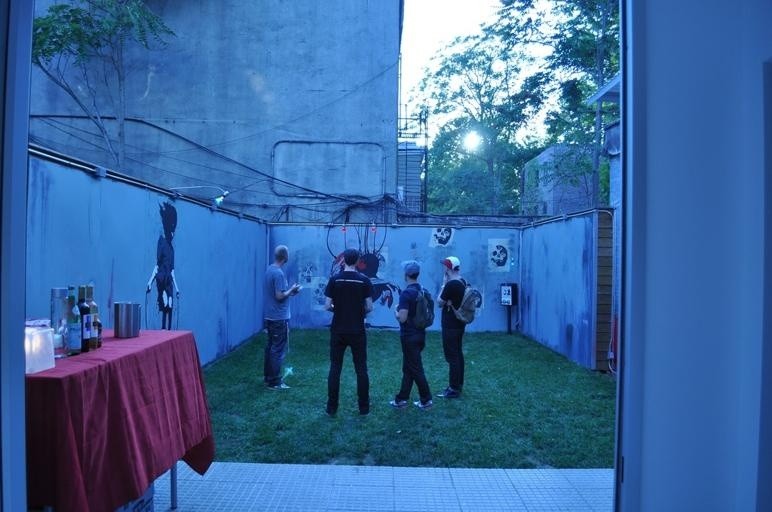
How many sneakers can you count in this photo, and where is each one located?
[435,388,460,400]
[389,399,408,410]
[413,400,434,410]
[358,408,372,418]
[266,380,292,393]
[323,408,339,419]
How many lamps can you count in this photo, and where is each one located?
[170,186,229,206]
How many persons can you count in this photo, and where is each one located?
[436,257,472,398]
[263,245,301,392]
[388,261,433,409]
[324,249,374,418]
[146,202,180,330]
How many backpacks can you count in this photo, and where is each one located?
[445,278,480,324]
[407,284,434,331]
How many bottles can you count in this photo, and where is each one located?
[51,283,102,357]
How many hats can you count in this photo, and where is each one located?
[441,256,460,271]
[400,260,421,274]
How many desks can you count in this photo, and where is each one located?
[25,329,215,512]
